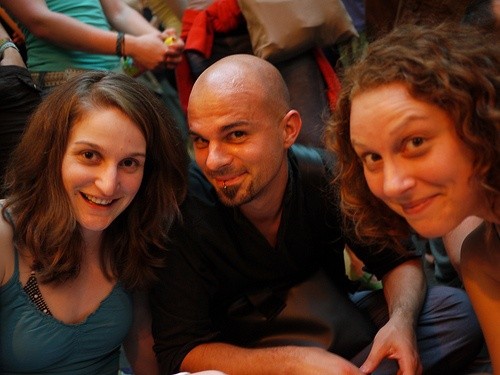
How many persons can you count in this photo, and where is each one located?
[322,21,499,375]
[0,70,187,375]
[0,0,500,375]
[151,53,493,375]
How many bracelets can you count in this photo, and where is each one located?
[116,32,123,58]
[122,33,127,60]
[0,42,19,56]
[0,37,14,46]
